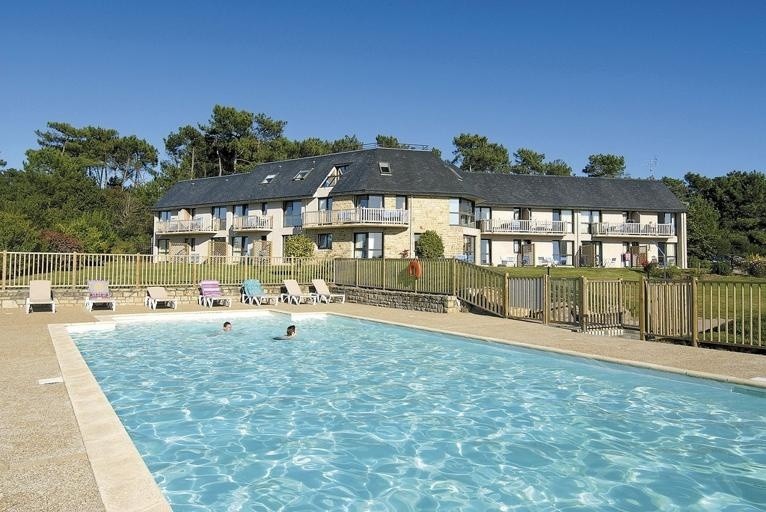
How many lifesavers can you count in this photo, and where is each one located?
[408,262,421,280]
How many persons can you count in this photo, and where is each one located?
[214,322,232,335]
[278,325,296,339]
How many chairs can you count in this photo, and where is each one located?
[24,279,55,314]
[83,278,347,312]
